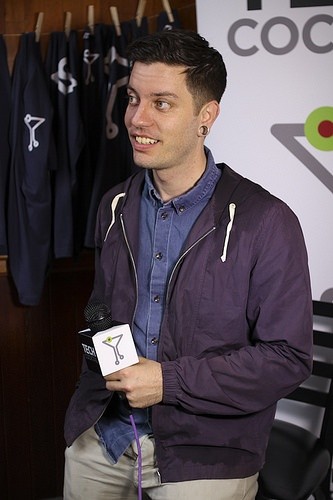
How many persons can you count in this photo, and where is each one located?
[63,29,314,500]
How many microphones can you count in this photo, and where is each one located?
[78,300,140,416]
